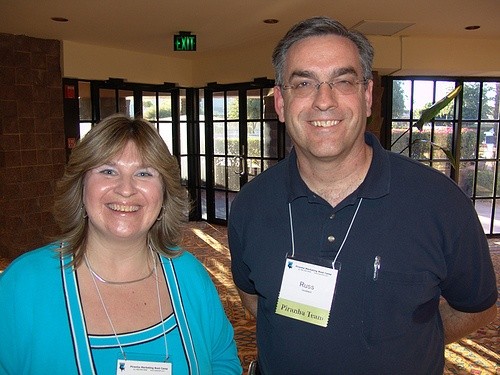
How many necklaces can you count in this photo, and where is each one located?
[81,251,157,285]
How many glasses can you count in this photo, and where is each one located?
[279,77,368,98]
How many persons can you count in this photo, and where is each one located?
[228,15,498,375]
[0,114,247,375]
